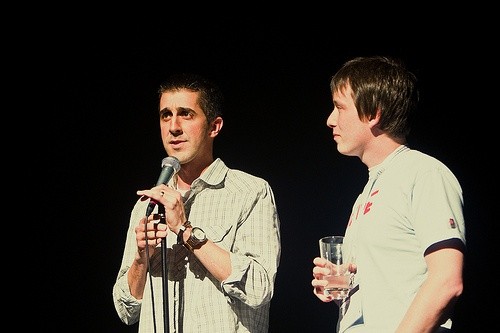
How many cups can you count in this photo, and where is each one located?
[318,236,351,299]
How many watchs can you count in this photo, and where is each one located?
[182,227,207,250]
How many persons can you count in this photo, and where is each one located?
[111,70,282,333]
[310,56,465,333]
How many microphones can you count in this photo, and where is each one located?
[145,156,182,216]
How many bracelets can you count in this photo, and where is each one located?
[177,221,191,245]
